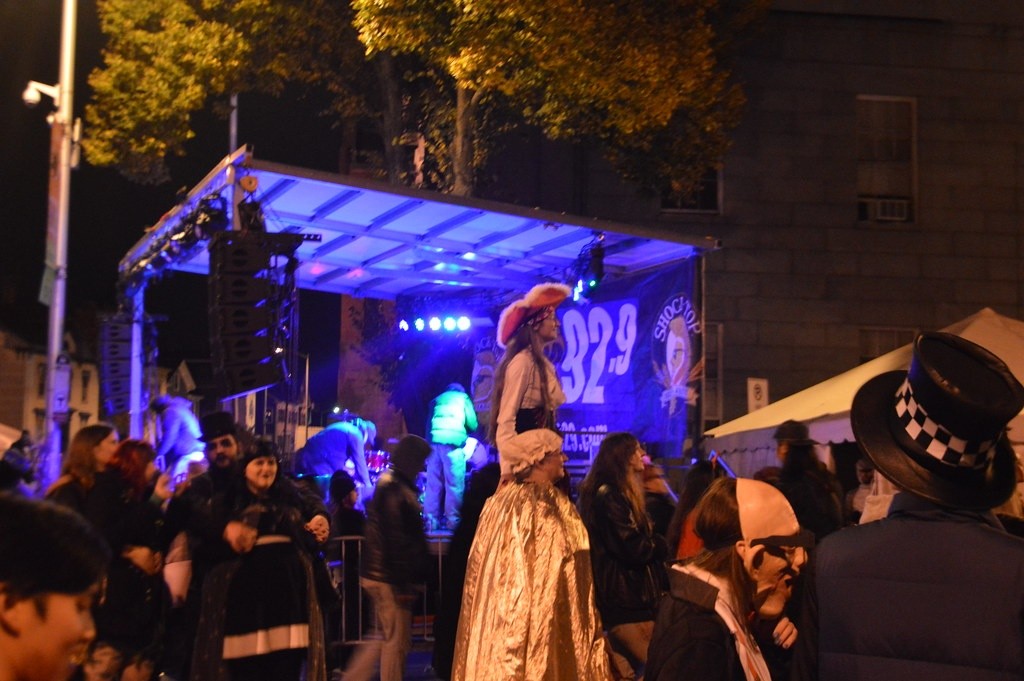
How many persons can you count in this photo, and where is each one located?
[452,428,615,681]
[577,419,842,681]
[794,331,1024,681]
[422,382,488,531]
[0,398,376,681]
[643,477,808,681]
[486,284,574,496]
[341,433,434,681]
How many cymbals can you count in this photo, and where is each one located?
[325,412,358,419]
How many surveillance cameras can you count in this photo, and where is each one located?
[23,88,40,109]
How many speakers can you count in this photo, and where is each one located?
[95,321,133,416]
[207,231,284,402]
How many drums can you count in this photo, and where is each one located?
[364,449,390,474]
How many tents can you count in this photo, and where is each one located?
[705,306,1023,478]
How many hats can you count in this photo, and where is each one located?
[851,331,1024,510]
[773,420,821,447]
[238,441,286,462]
[498,284,574,348]
[195,411,242,442]
[329,477,356,499]
[501,428,562,475]
